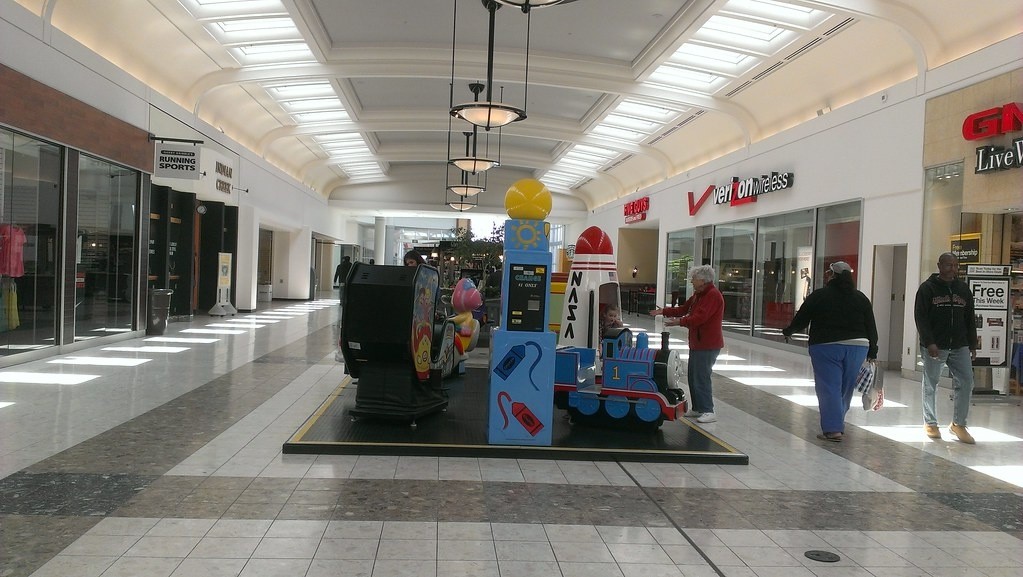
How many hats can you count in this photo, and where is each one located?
[830,261,853,274]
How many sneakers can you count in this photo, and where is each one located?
[685,410,702,417]
[949,422,975,444]
[697,412,716,423]
[923,424,941,438]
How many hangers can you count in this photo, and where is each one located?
[0,278,18,287]
[0,216,18,226]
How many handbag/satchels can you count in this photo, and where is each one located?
[855,359,884,410]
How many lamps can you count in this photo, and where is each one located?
[936,167,944,180]
[445,160,479,212]
[943,165,952,178]
[494,0,577,9]
[952,164,959,177]
[631,265,638,279]
[446,128,487,197]
[446,83,504,175]
[447,0,530,129]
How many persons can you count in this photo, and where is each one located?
[599,305,624,356]
[334,255,352,308]
[914,252,978,445]
[782,261,879,442]
[649,265,725,424]
[670,275,683,308]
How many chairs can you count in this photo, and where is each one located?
[556,346,598,388]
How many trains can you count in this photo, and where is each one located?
[555,327,688,430]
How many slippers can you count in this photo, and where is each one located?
[817,434,840,442]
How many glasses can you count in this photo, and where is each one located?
[942,262,961,269]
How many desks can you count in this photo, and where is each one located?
[639,288,656,312]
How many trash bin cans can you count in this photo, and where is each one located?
[146,289,174,335]
